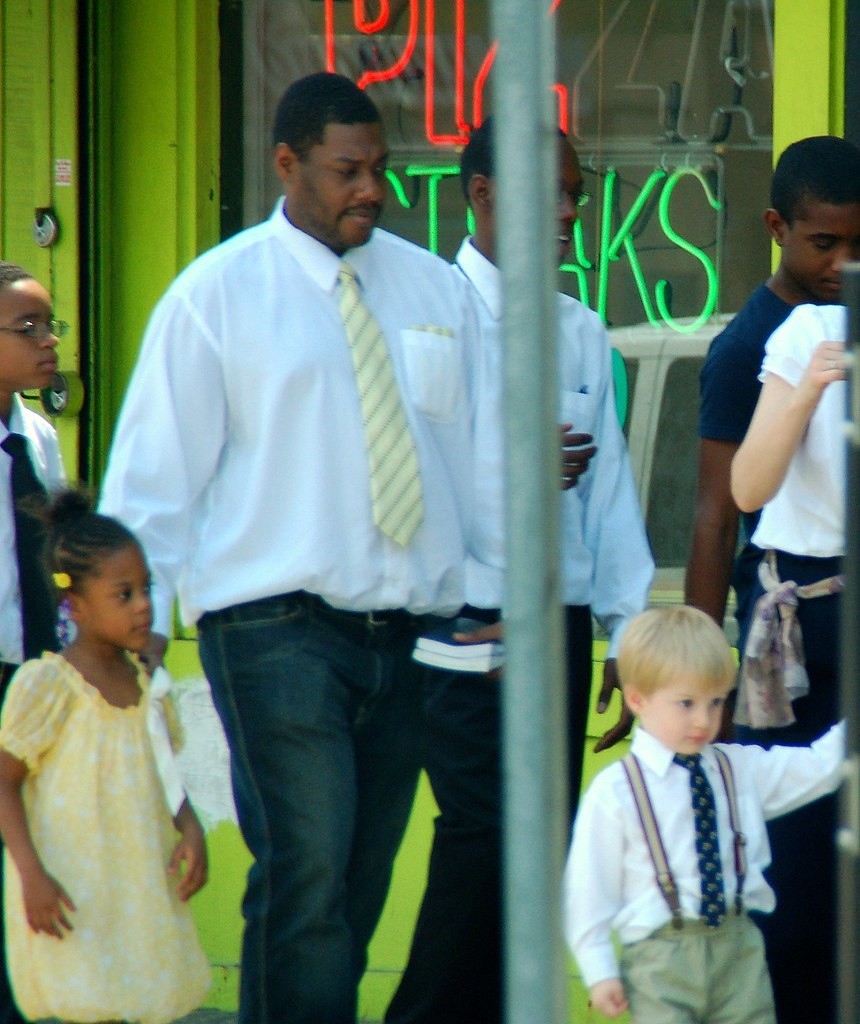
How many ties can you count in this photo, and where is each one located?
[337,261,424,548]
[0,433,64,662]
[672,752,726,931]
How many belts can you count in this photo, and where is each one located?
[268,589,412,626]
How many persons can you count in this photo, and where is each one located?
[0,490,213,1024]
[565,606,845,1024]
[728,303,849,1024]
[675,135,860,654]
[0,258,78,710]
[382,113,657,1024]
[94,73,505,1024]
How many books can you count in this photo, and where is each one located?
[412,617,505,674]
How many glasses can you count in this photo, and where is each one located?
[0,320,70,338]
[557,188,593,207]
